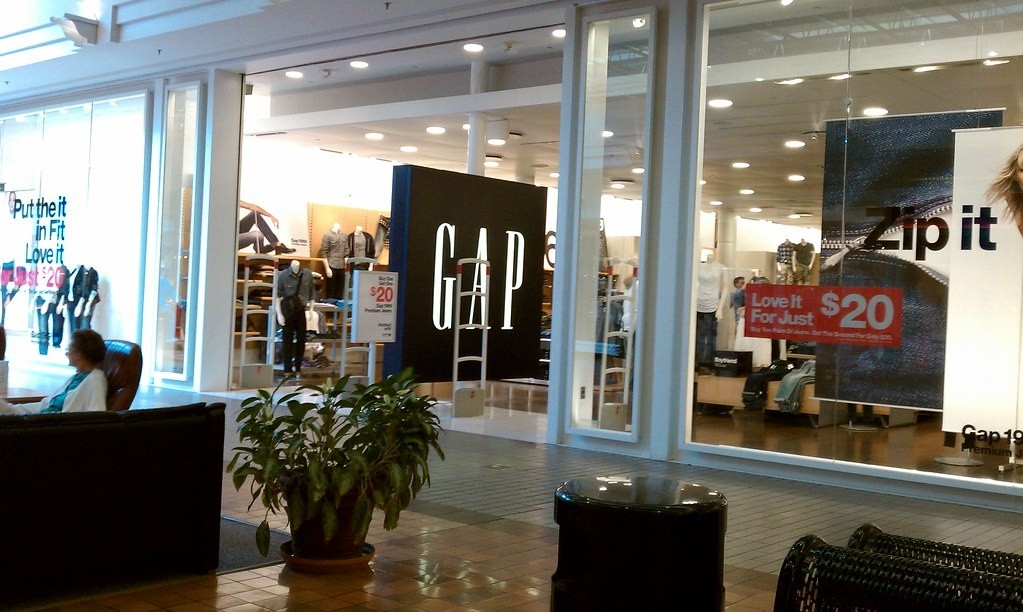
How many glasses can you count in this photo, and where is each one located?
[66,347,70,352]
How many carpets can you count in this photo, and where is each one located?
[22,515,292,612]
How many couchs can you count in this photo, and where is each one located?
[0,401,226,612]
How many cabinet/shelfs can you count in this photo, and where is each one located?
[500,337,551,386]
[543,268,619,317]
[234,252,389,349]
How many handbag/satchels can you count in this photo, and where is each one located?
[276,273,306,326]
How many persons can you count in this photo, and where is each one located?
[347,225,376,287]
[35,262,100,355]
[792,238,816,287]
[239,200,296,254]
[0,329,107,411]
[777,239,796,286]
[275,259,316,375]
[321,224,350,324]
[732,273,755,338]
[696,254,729,373]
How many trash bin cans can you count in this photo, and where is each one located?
[550,474,728,612]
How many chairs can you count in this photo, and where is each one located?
[94,340,143,411]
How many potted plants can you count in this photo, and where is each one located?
[224,365,446,575]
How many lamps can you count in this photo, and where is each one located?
[486,119,510,145]
